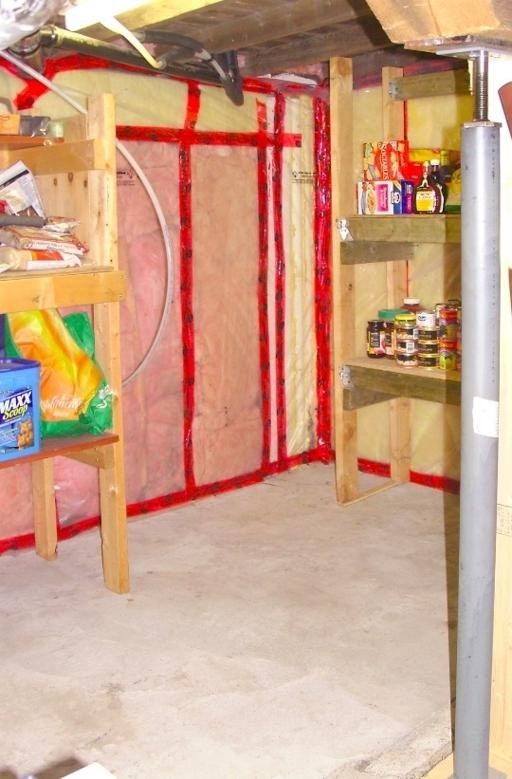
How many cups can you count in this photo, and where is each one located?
[46,121,64,145]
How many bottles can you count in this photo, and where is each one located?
[415,149,454,214]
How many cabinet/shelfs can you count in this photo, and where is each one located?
[0,91,130,594]
[329,56,475,507]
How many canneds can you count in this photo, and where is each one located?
[366,304,462,367]
